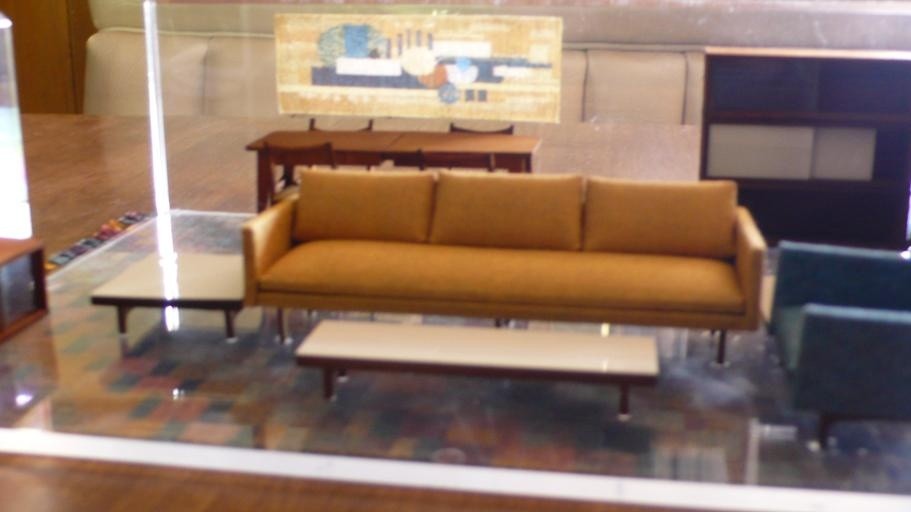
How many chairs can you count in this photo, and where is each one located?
[768,240,911,450]
[244,119,543,214]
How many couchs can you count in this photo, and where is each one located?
[240,170,768,372]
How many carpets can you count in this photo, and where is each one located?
[1,210,911,493]
